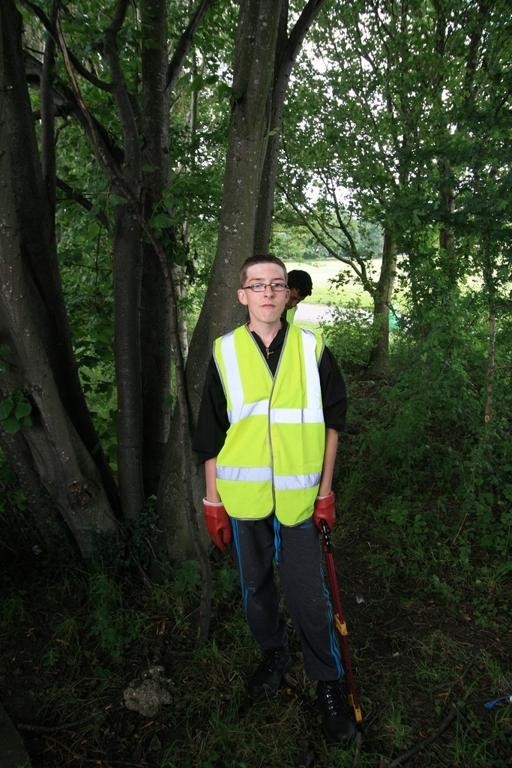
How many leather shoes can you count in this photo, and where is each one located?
[248,645,294,702]
[315,680,355,744]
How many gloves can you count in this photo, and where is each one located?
[203,496,232,551]
[313,491,337,533]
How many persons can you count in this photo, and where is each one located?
[190,252,359,747]
[285,267,314,324]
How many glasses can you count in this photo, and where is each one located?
[242,282,289,292]
